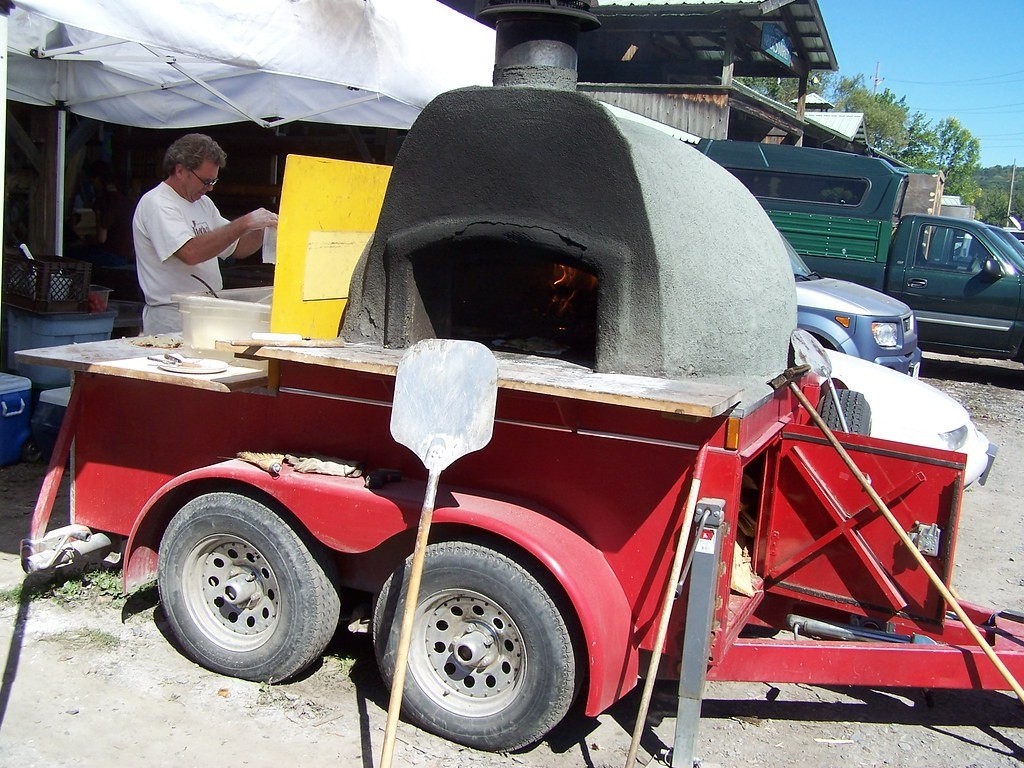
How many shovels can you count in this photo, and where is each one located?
[368,334,500,768]
[789,326,851,433]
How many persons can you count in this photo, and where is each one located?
[131,133,278,335]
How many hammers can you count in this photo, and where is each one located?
[767,362,1024,722]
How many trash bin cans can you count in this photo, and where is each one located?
[5,309,120,404]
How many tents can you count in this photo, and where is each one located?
[7,1,496,129]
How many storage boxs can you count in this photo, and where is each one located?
[6,307,118,384]
[2,254,92,315]
[31,387,70,469]
[0,371,44,466]
[171,286,272,367]
[91,284,114,313]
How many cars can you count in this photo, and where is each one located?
[775,221,922,383]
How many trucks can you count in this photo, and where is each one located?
[695,137,1024,365]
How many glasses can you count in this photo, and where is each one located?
[187,166,219,186]
